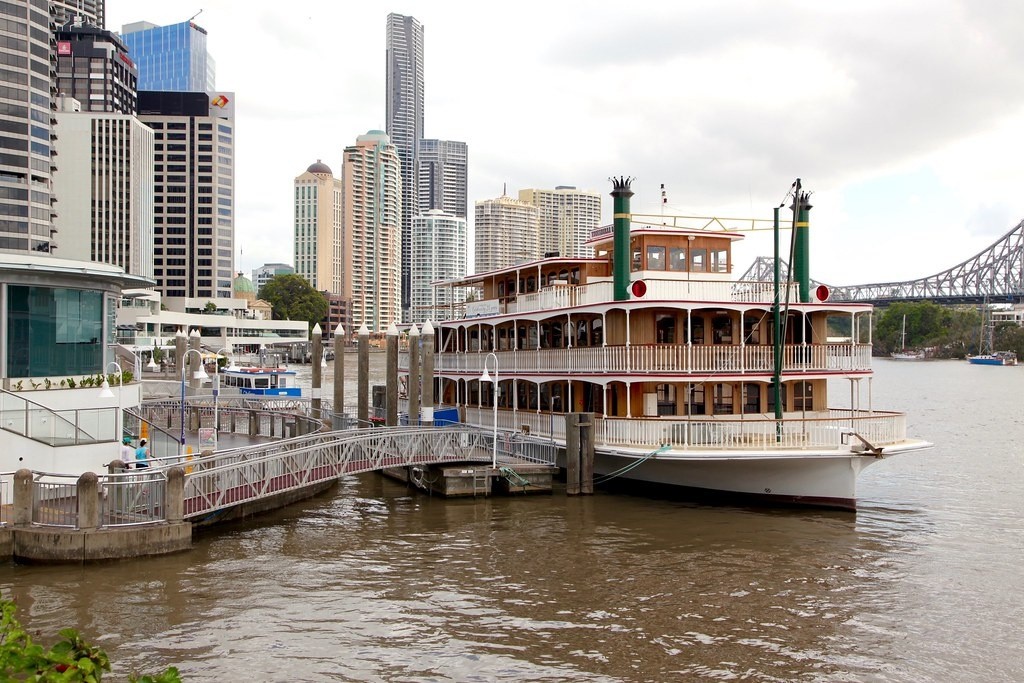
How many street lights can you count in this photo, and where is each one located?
[478,352,499,470]
[212,347,241,452]
[137,345,158,441]
[97,361,123,462]
[179,348,210,461]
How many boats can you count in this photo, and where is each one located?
[270,340,334,363]
[362,173,938,517]
[219,347,303,411]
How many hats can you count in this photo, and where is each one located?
[141,438,146,443]
[123,438,131,443]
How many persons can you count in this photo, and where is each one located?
[122,438,133,469]
[134,438,152,494]
[499,390,559,410]
